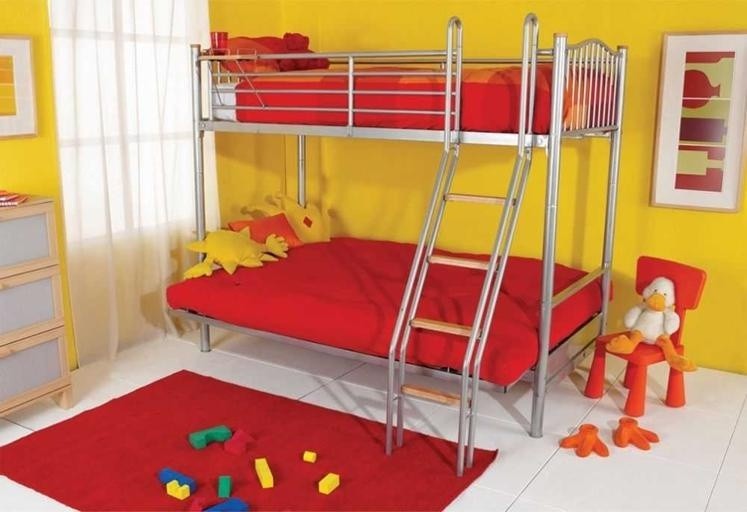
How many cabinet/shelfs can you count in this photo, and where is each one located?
[0,192,79,426]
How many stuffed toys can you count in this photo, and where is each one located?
[603,273,695,374]
[557,423,609,457]
[183,225,288,281]
[241,190,333,244]
[611,417,659,450]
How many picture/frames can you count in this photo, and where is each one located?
[1,32,38,141]
[649,32,747,215]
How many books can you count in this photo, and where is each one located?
[0,189,31,207]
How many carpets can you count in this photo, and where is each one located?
[0,370,496,512]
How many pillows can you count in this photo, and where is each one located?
[183,197,334,278]
[218,31,329,71]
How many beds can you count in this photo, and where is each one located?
[164,13,628,477]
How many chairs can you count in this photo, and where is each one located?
[583,255,707,416]
